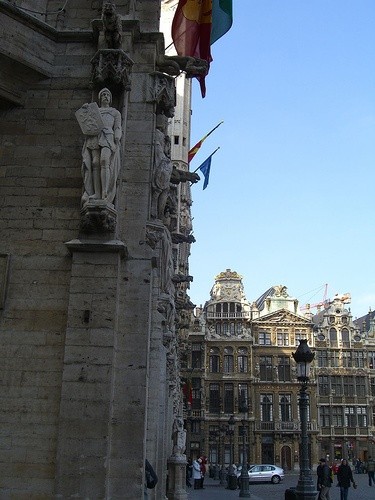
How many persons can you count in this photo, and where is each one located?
[317,458,332,500]
[352,457,367,474]
[366,456,375,487]
[336,459,355,500]
[75,87,122,201]
[186,456,208,489]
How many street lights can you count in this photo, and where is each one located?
[292,338,320,500]
[237,388,251,498]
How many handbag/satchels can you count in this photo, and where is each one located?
[353,483,357,489]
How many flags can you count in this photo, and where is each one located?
[171,0,233,97]
[188,120,224,164]
[183,373,193,408]
[193,147,220,190]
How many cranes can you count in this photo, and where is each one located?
[299,282,352,311]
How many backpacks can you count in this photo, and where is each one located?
[328,467,334,483]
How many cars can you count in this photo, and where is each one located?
[237,463,285,484]
